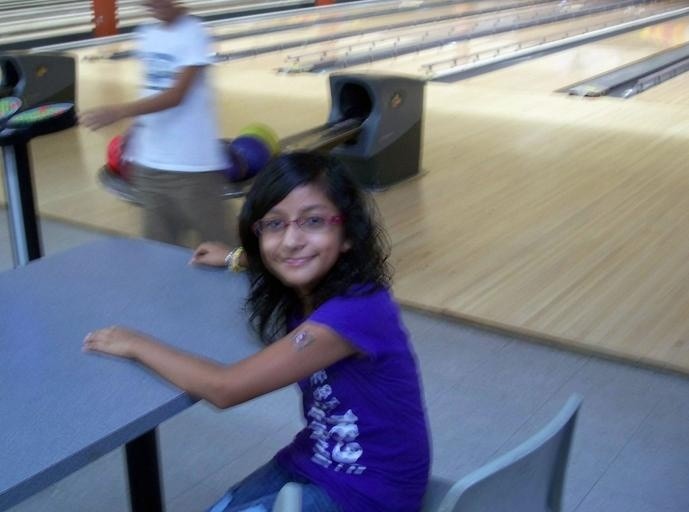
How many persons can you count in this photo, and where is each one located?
[73,0,230,256]
[81,150,430,512]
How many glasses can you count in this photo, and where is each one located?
[253,214,341,238]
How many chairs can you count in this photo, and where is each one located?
[382,390,581,511]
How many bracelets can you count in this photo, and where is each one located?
[224,246,249,273]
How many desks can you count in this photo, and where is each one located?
[0,229,309,512]
